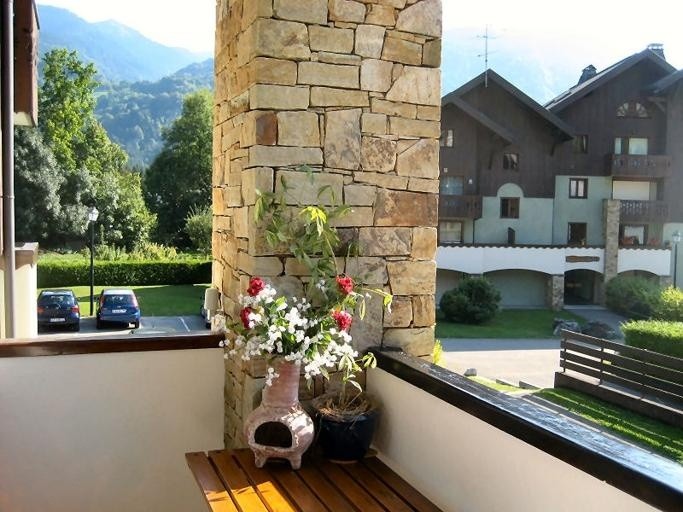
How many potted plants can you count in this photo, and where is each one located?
[254,165,393,462]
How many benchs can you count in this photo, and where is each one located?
[185,449,444,512]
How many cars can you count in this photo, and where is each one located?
[37,288,80,333]
[92,288,141,329]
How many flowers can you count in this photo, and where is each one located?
[209,275,359,387]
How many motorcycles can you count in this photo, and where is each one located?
[199,296,204,318]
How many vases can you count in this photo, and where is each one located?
[243,356,315,471]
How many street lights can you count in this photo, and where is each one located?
[88,207,100,319]
[672,229,681,290]
